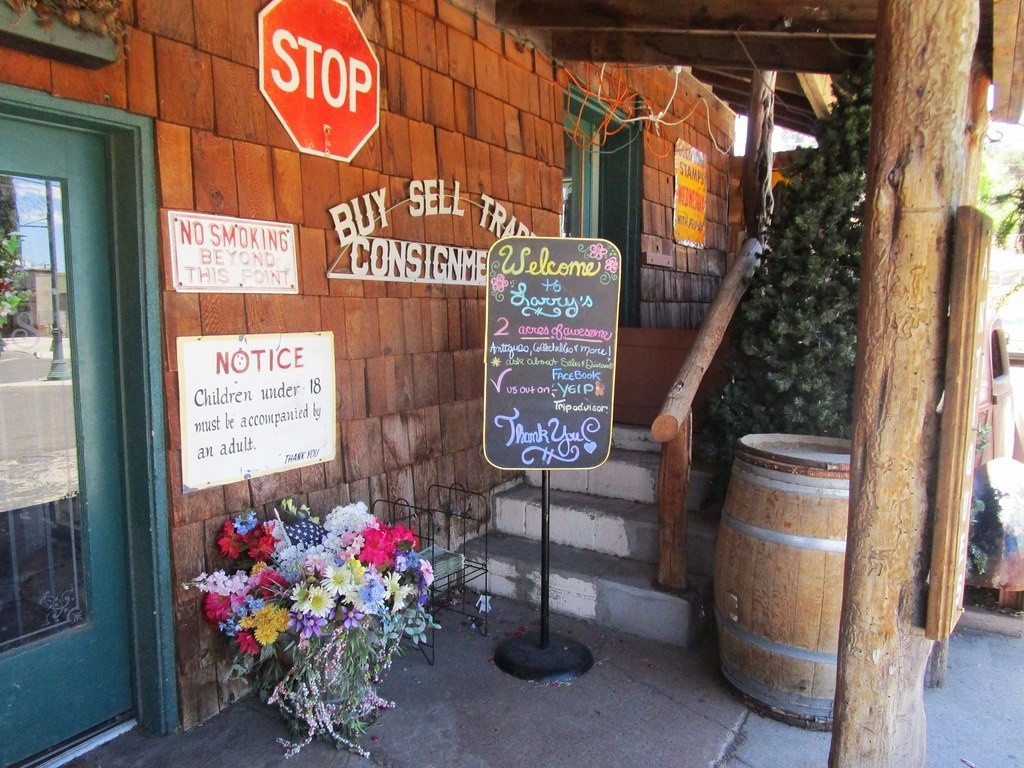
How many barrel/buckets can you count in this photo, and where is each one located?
[715,431,851,729]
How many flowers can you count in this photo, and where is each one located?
[180,498,442,760]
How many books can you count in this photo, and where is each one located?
[415,543,465,581]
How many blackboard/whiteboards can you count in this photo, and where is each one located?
[482,236,622,471]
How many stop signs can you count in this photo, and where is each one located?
[256,0,380,164]
